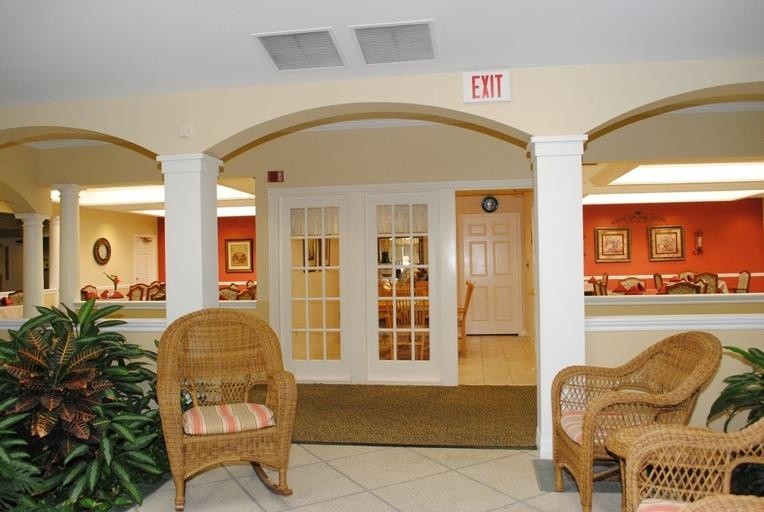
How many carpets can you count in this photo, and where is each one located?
[291,383,537,450]
[533,459,622,493]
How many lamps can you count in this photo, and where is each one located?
[693,232,703,256]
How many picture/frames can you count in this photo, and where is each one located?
[224,238,254,274]
[303,239,330,273]
[378,236,423,265]
[595,226,632,263]
[648,224,686,261]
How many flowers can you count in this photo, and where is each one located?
[103,272,120,290]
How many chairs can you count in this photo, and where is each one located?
[157,307,298,512]
[551,331,722,512]
[583,270,751,296]
[376,280,474,361]
[625,417,763,511]
[0,280,258,304]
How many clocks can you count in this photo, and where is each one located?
[481,196,498,213]
[94,238,111,265]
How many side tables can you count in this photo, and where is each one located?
[605,423,726,512]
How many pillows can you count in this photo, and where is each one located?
[182,403,275,435]
